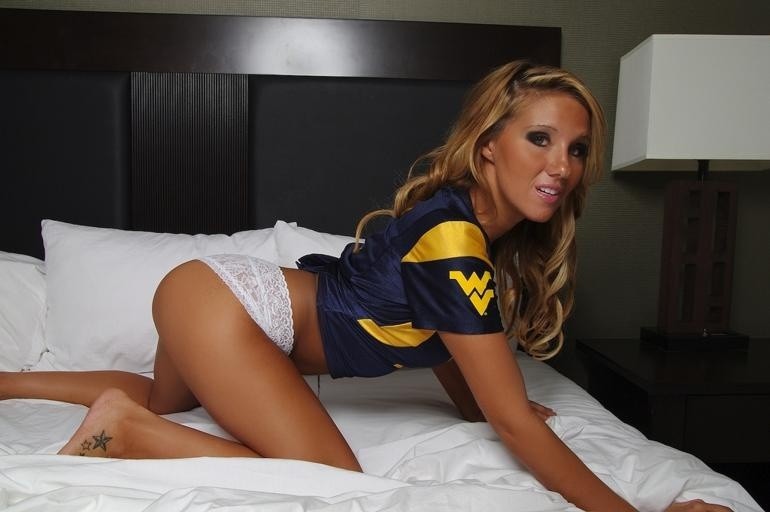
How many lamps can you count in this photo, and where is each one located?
[611,33,770,352]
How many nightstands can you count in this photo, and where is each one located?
[575,334,770,512]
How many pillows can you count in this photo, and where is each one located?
[37,217,297,374]
[1,250,46,371]
[270,220,370,272]
